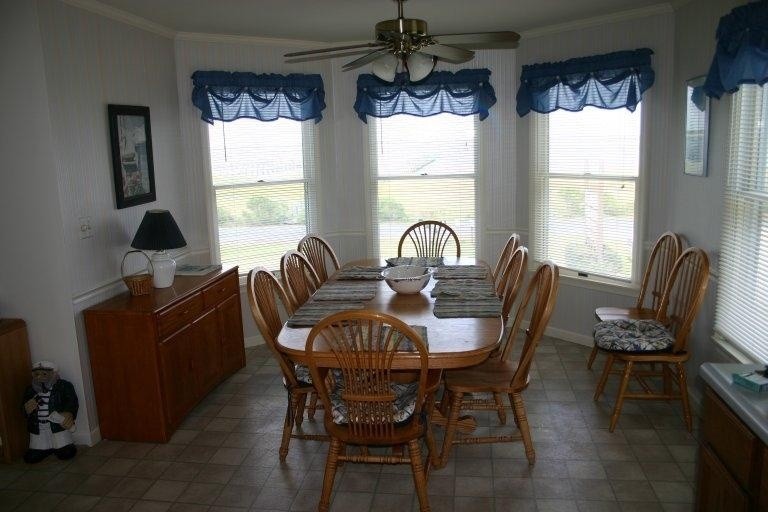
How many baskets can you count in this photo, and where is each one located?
[120,250,156,298]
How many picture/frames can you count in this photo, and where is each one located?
[108,104,156,210]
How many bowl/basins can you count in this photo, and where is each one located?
[380,264,434,294]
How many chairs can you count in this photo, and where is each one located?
[492,234,521,286]
[396,220,462,261]
[298,234,342,291]
[496,246,528,319]
[595,246,711,434]
[587,230,684,402]
[279,248,332,421]
[305,309,440,511]
[247,264,420,465]
[441,261,560,467]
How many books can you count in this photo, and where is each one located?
[175,265,221,276]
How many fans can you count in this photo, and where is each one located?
[284,0,520,74]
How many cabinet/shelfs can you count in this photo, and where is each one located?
[698,361,768,512]
[83,265,247,444]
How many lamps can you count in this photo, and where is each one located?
[372,0,437,85]
[131,208,188,289]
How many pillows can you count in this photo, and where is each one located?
[592,318,675,353]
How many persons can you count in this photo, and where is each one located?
[18,362,79,463]
